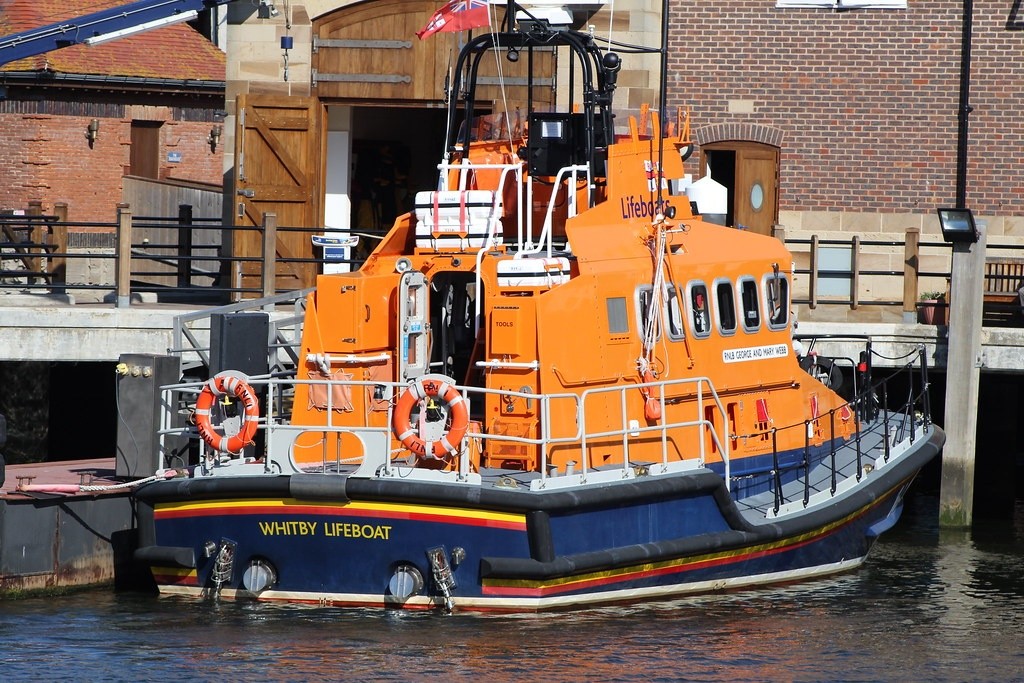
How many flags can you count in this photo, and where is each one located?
[415,0,492,41]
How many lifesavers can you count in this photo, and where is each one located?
[194,376,260,454]
[392,379,470,459]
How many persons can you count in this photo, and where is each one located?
[1018,276,1024,314]
[465,287,484,353]
[351,144,435,245]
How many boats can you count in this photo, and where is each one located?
[121,0,949,618]
